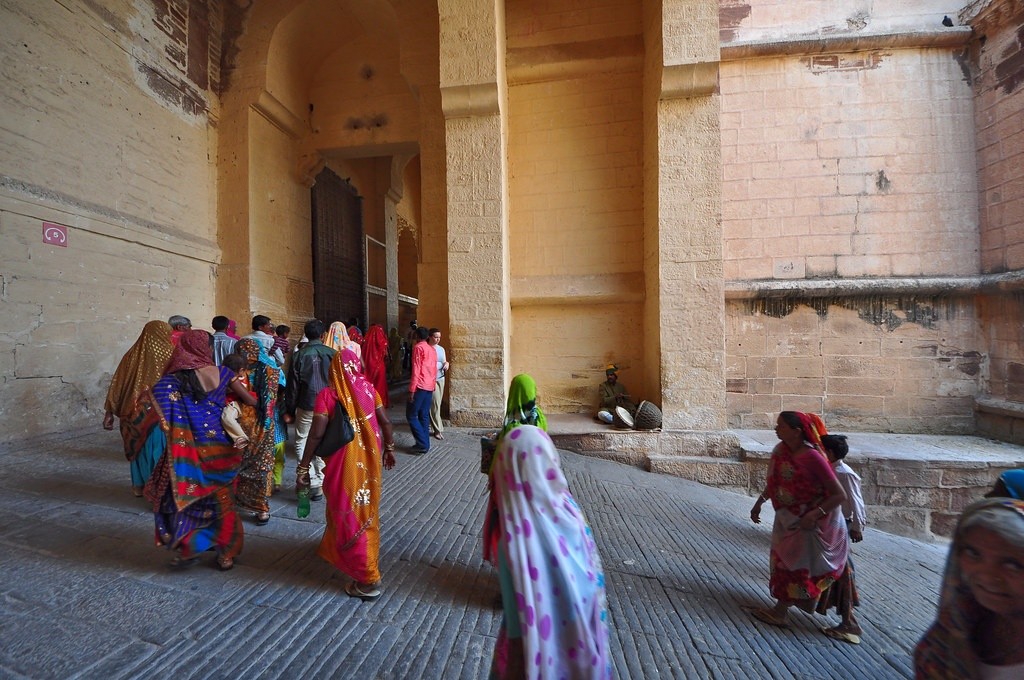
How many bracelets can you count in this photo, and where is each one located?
[761,495,766,502]
[385,443,394,451]
[818,507,826,514]
[296,464,311,474]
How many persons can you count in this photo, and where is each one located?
[487,425,614,680]
[482,375,547,566]
[296,349,396,573]
[233,337,281,526]
[406,326,438,453]
[103,320,174,496]
[325,321,417,408]
[212,315,309,492]
[221,354,251,449]
[750,411,866,644]
[984,469,1024,500]
[912,496,1024,680]
[168,316,192,345]
[597,365,636,423]
[287,319,339,501]
[427,328,450,439]
[143,328,256,570]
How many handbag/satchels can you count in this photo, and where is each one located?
[313,392,354,458]
[479,434,499,473]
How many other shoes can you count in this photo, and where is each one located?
[216,554,233,570]
[308,486,324,502]
[274,483,281,493]
[169,553,202,569]
[131,486,144,496]
[407,447,428,455]
[433,431,445,440]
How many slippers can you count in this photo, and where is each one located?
[821,626,861,644]
[753,608,793,628]
[254,510,270,525]
[344,580,380,599]
